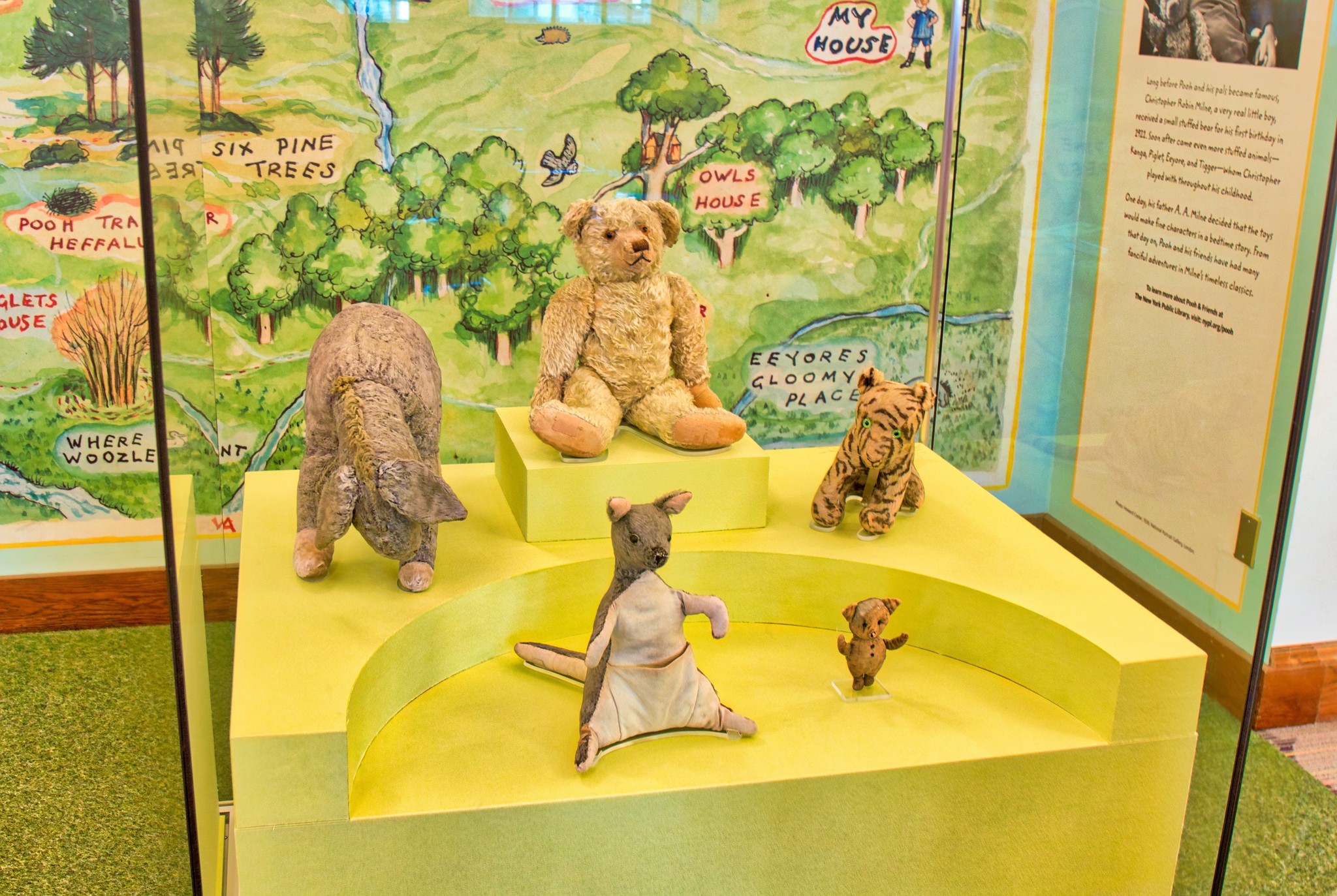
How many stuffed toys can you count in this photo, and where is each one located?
[810,366,934,534]
[527,195,744,456]
[838,597,908,690]
[294,303,468,593]
[512,490,759,771]
